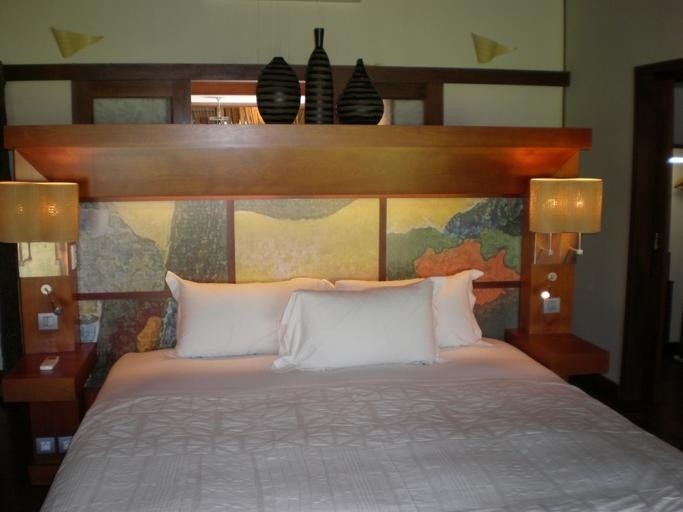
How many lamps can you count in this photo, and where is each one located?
[666,147,682,164]
[1,183,81,267]
[40,284,63,315]
[528,177,606,265]
[539,272,558,301]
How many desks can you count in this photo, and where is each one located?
[502,331,611,382]
[0,341,99,486]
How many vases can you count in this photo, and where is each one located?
[303,27,334,124]
[336,58,385,125]
[255,57,301,124]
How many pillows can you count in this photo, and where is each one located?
[272,276,442,371]
[334,267,485,351]
[163,269,336,361]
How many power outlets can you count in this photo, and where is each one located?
[58,434,73,456]
[33,437,54,456]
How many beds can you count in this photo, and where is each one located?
[39,338,683,512]
